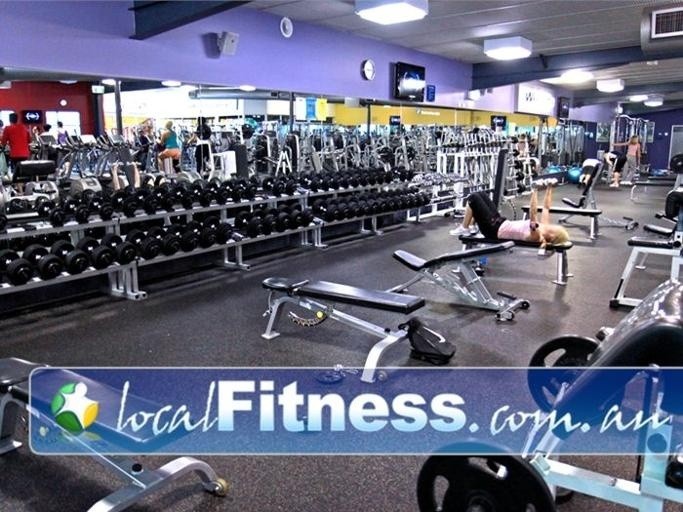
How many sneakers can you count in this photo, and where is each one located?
[449,224,472,237]
[609,178,633,188]
[468,224,478,234]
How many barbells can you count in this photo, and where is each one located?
[442,132,538,196]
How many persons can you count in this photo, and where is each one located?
[1,113,32,198]
[38,124,56,145]
[56,121,66,145]
[135,117,153,171]
[612,134,640,182]
[154,120,181,172]
[601,151,627,188]
[578,158,601,189]
[449,178,570,243]
[190,117,211,181]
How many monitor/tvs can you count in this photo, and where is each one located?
[0,110,16,129]
[558,97,570,118]
[45,111,80,139]
[394,61,426,102]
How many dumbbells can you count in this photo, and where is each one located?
[414,171,467,188]
[0,166,431,287]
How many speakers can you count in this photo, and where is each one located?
[215,30,240,56]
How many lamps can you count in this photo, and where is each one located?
[354,0,429,26]
[482,36,533,63]
[643,96,663,107]
[595,79,625,93]
